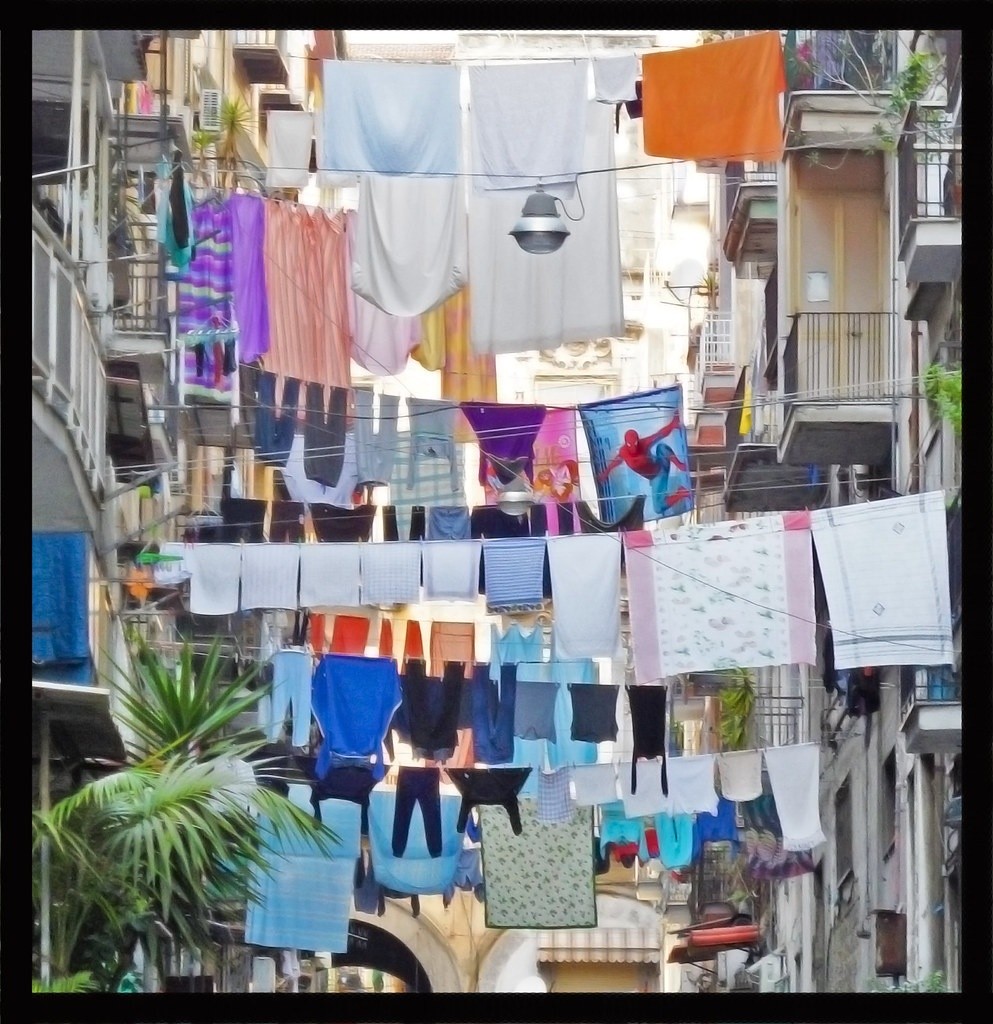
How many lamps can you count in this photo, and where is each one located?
[507,177,572,256]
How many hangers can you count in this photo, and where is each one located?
[186,302,239,346]
[136,524,185,572]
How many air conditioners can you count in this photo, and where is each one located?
[200,88,228,132]
